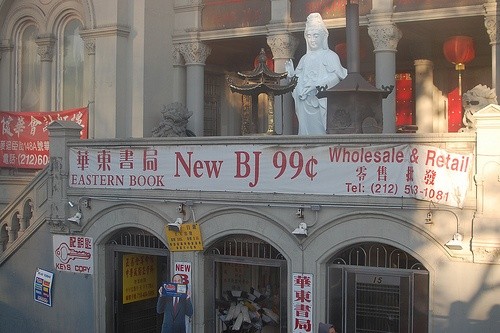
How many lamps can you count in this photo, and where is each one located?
[291,223,307,237]
[168,217,183,232]
[67,212,81,224]
[445,233,463,250]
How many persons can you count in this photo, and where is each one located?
[284,12,349,137]
[156,274,194,333]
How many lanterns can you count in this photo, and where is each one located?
[252,51,275,72]
[331,34,367,69]
[440,33,476,98]
